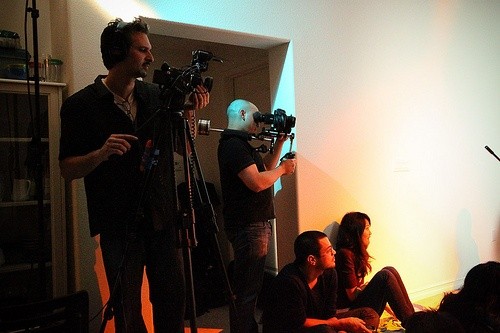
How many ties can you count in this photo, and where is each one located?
[122,100,135,122]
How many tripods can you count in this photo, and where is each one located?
[99,97,242,333]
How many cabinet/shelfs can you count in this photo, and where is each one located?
[0,78,68,324]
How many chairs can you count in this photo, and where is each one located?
[0,289,90,333]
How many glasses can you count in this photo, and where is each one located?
[319,246,333,258]
[115,20,130,32]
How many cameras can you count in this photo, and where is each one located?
[153,50,224,97]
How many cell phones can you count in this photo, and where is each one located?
[366,325,376,333]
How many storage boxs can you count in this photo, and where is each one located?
[0,58,29,79]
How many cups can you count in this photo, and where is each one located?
[12,178,31,201]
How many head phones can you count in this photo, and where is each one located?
[110,22,128,64]
